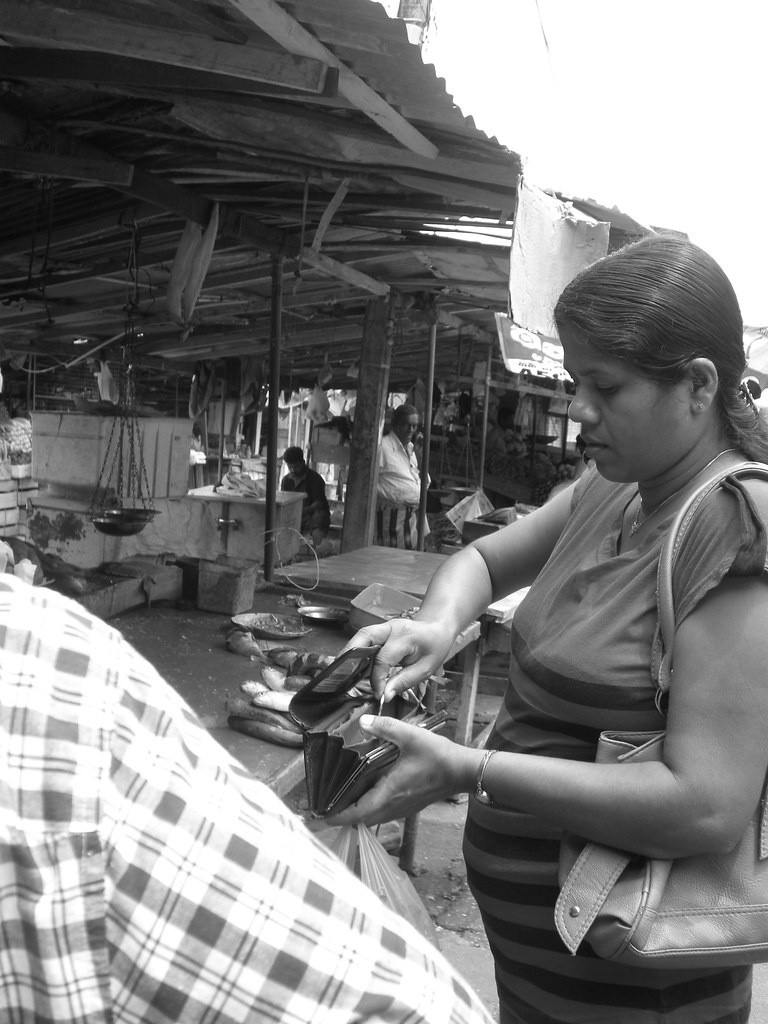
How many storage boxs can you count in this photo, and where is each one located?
[196,553,259,615]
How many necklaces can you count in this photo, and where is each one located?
[629,449,737,535]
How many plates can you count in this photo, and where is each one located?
[297,605,350,625]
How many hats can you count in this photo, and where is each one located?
[284,447,303,464]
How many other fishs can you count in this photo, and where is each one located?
[221,628,335,746]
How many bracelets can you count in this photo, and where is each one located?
[475,750,497,808]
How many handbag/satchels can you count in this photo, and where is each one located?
[554,450,768,966]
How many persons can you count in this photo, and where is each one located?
[325,233,767,1024]
[0,571,500,1023]
[280,445,331,554]
[374,403,433,552]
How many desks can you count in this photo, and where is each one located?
[188,485,308,570]
[105,586,482,877]
[274,544,532,804]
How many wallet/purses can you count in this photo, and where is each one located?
[289,644,447,819]
